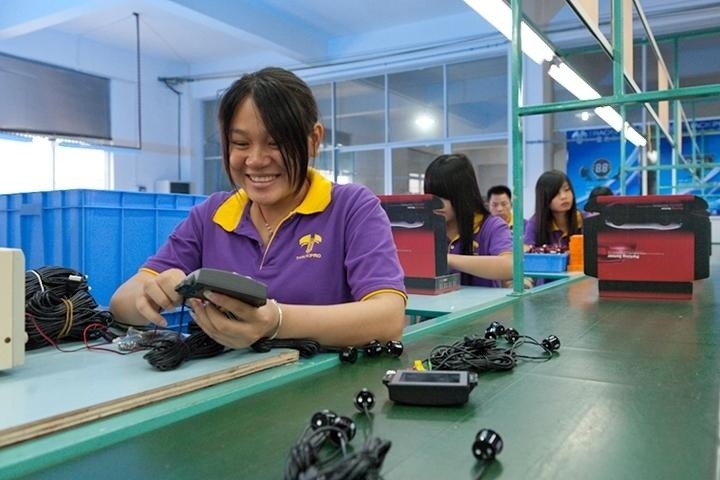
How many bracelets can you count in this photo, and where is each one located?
[266,298,283,341]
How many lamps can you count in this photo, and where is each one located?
[593,106,623,132]
[624,125,648,147]
[547,63,601,100]
[463,0,557,66]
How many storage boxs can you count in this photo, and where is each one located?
[1,191,207,335]
[523,251,568,273]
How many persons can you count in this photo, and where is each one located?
[108,67,408,348]
[424,153,613,289]
